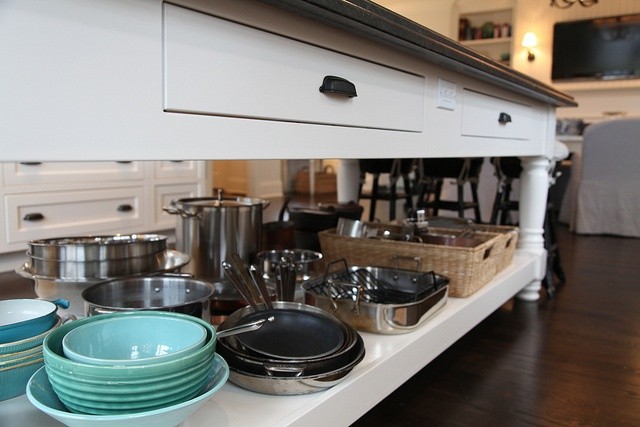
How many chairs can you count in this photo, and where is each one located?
[490,152,575,300]
[357,159,411,218]
[416,157,484,223]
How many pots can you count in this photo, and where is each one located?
[162,186,261,291]
[255,249,323,292]
[81,273,215,311]
[217,261,366,396]
[257,195,295,244]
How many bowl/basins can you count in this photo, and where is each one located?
[15,233,195,319]
[0,298,62,402]
[26,311,227,427]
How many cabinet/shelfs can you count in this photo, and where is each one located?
[0,0,578,427]
[456,7,513,45]
[3,160,205,258]
